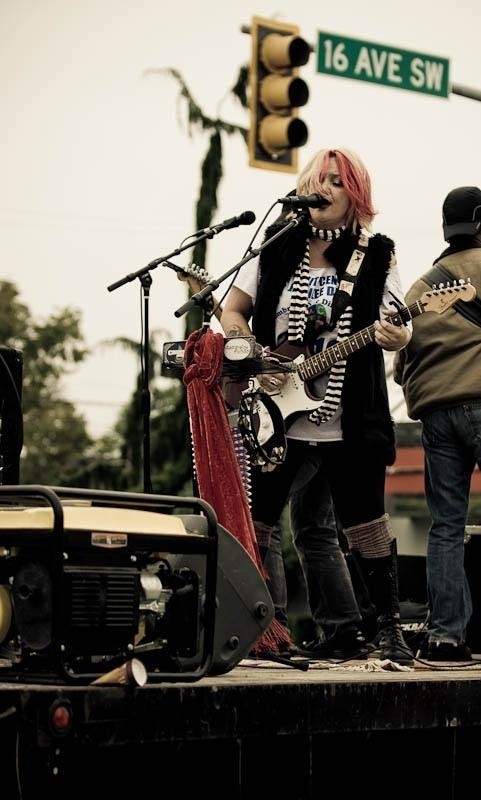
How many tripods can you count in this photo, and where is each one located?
[175,213,309,670]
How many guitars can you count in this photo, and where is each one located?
[217,278,476,459]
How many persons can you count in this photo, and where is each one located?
[392,184,481,661]
[262,186,371,664]
[219,148,417,667]
[221,374,371,661]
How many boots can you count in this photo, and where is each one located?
[348,538,415,666]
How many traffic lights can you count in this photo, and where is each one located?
[246,14,314,176]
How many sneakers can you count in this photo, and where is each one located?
[427,634,458,649]
[295,624,367,660]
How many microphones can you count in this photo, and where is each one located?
[278,193,324,208]
[195,211,255,238]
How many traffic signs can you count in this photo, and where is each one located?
[316,27,453,100]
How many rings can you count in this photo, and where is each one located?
[270,377,279,385]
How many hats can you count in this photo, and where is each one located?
[442,187,480,241]
